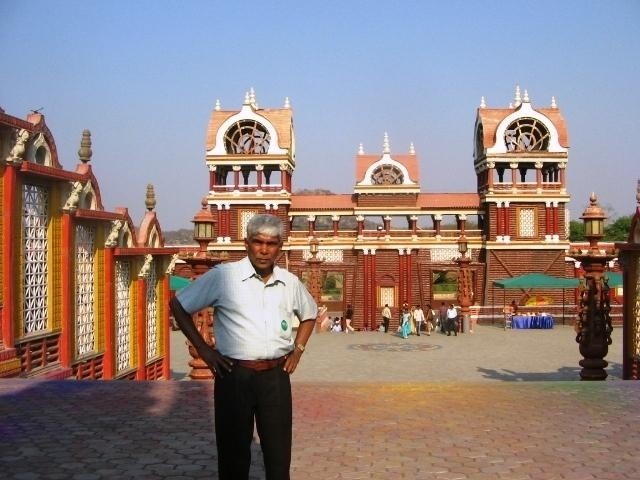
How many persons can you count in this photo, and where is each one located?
[166,211,320,480]
[327,301,460,340]
[509,300,518,315]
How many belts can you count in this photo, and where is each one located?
[238,355,288,372]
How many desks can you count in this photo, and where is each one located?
[511,314,555,329]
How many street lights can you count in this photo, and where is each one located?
[565,187,619,381]
[303,234,325,332]
[179,196,215,379]
[453,230,478,334]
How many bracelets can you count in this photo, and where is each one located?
[295,342,306,353]
